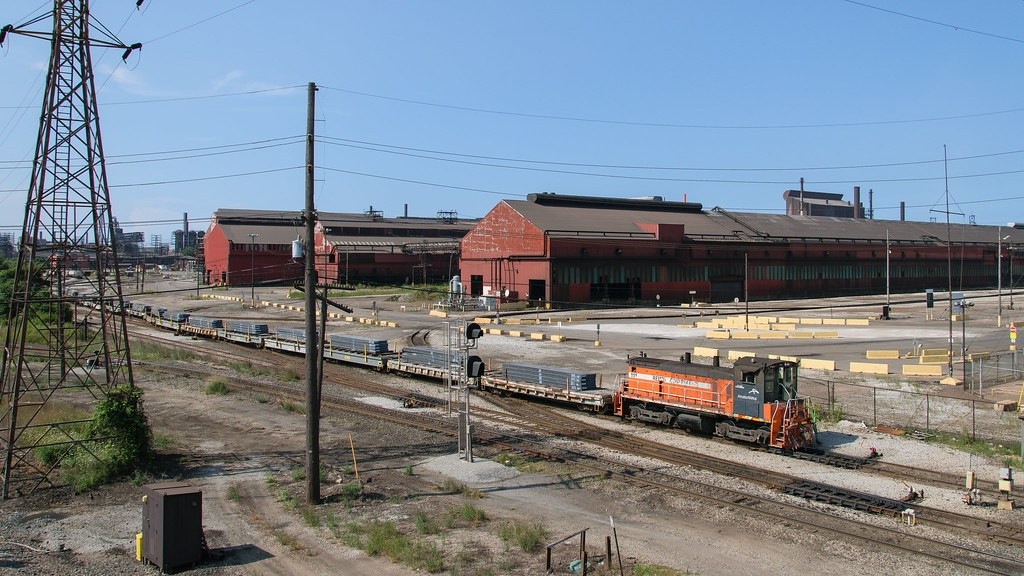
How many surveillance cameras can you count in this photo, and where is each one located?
[453,275,460,293]
[291,234,302,260]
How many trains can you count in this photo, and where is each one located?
[64,291,818,454]
